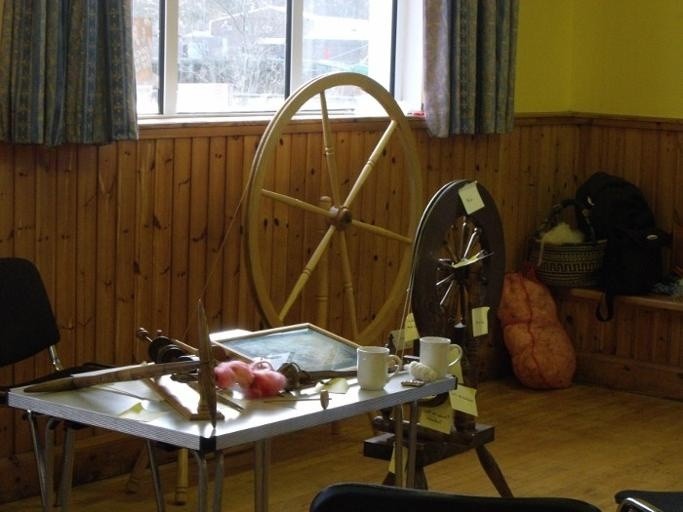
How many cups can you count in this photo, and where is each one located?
[418,336,460,380]
[355,345,403,393]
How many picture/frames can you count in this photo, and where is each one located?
[211,319,362,383]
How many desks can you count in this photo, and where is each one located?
[6,339,459,511]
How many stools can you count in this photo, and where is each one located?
[613,486,682,512]
[362,417,516,498]
[307,480,603,510]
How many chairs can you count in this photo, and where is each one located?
[0,255,169,511]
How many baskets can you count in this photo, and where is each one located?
[529,199,612,289]
